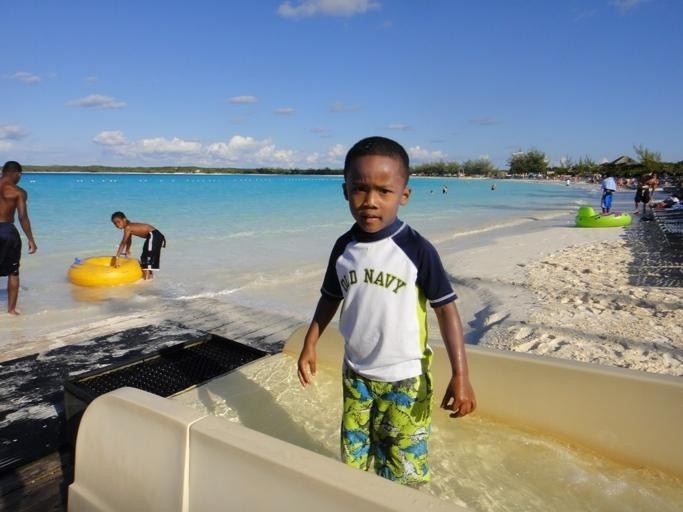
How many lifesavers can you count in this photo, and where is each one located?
[575,206,632,228]
[69,257,143,286]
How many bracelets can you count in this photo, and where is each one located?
[112,256,118,259]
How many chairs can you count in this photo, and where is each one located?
[649,199,683,247]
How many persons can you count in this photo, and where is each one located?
[0,162,37,316]
[293,136,478,495]
[442,185,448,195]
[491,184,496,190]
[633,170,659,214]
[600,172,616,213]
[108,212,167,280]
[647,180,683,209]
